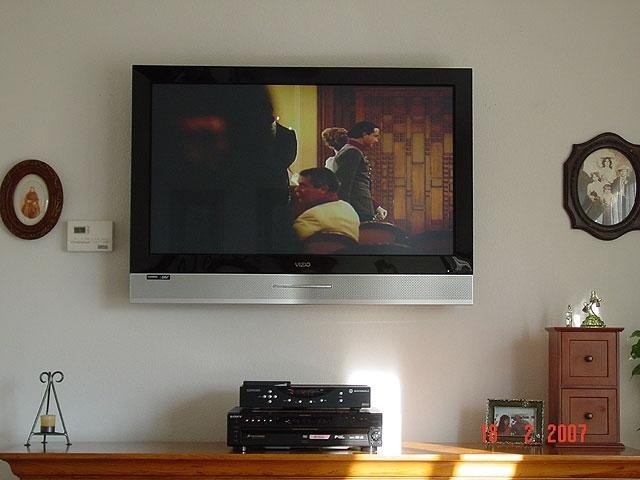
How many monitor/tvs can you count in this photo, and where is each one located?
[129,64,475,307]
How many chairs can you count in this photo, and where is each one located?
[295,217,453,255]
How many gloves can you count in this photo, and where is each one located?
[375,206,387,219]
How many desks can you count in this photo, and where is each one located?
[2,441,639,480]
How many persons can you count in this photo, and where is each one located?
[601,184,617,224]
[322,127,348,174]
[496,415,513,436]
[294,166,360,242]
[587,172,603,202]
[598,157,617,183]
[614,168,633,219]
[512,415,529,435]
[334,122,387,223]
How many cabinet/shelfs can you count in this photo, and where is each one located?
[544,325,625,453]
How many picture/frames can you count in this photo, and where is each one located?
[484,398,543,446]
[0,159,64,240]
[563,131,639,241]
